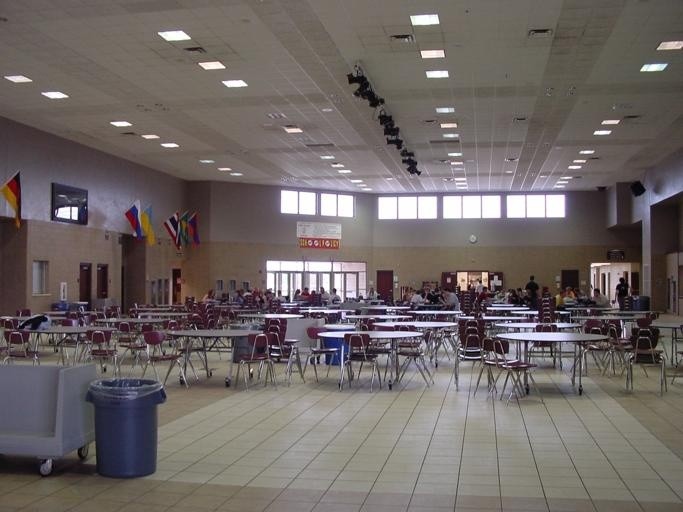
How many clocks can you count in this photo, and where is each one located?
[468,234,478,243]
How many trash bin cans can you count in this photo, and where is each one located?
[85,378,168,478]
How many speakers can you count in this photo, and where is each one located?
[629,182,645,196]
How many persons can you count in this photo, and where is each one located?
[367,287,377,301]
[509,289,523,306]
[554,290,562,306]
[276,290,286,303]
[466,279,476,292]
[293,287,302,302]
[478,286,493,302]
[301,287,310,298]
[319,285,330,303]
[405,284,445,306]
[564,286,576,300]
[475,277,486,293]
[308,289,316,305]
[442,287,459,308]
[526,275,540,310]
[331,286,342,305]
[615,278,629,311]
[202,286,275,310]
[591,288,607,304]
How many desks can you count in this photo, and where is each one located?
[317,330,423,390]
[2,325,119,366]
[167,328,265,387]
[493,330,609,396]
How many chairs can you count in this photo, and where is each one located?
[487,341,538,407]
[626,335,669,398]
[267,332,305,386]
[339,333,382,392]
[89,330,124,379]
[234,334,277,391]
[395,337,435,388]
[1,331,40,366]
[302,326,340,383]
[454,333,499,395]
[139,331,189,393]
[473,338,521,395]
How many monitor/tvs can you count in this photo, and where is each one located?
[562,270,579,288]
[51,182,88,225]
